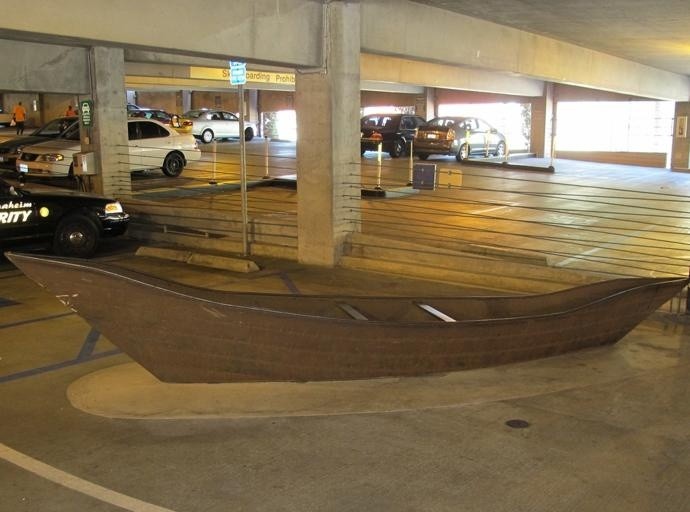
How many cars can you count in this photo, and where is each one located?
[358,113,508,163]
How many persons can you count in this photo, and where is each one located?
[11,101,26,136]
[66,105,74,117]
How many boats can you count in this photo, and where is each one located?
[0,243,689,389]
